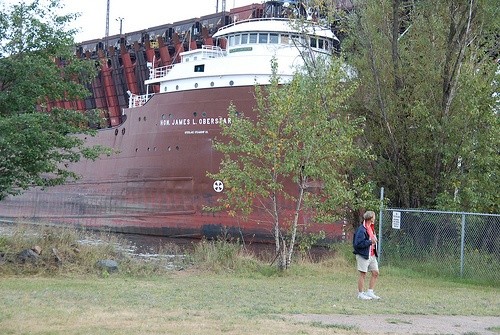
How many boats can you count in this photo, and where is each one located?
[0,0,359,247]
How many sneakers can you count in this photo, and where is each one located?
[366,291,380,300]
[358,292,372,300]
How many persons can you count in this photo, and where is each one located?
[301,3,318,21]
[281,2,300,19]
[353,210,381,299]
